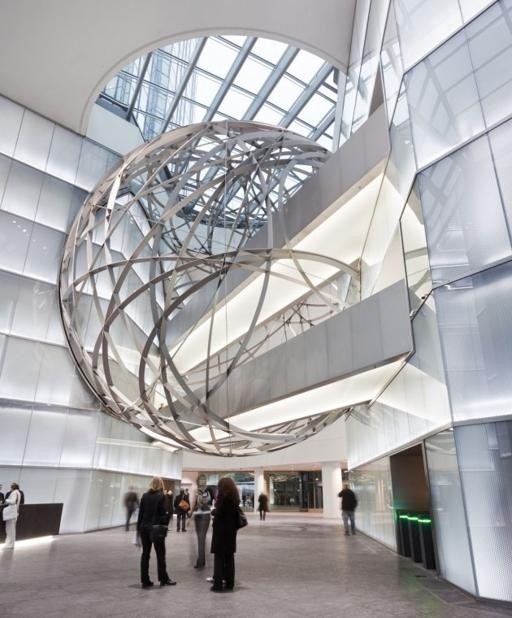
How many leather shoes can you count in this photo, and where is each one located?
[160,579,176,586]
[143,581,154,588]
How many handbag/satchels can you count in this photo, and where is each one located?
[178,500,190,511]
[235,505,247,530]
[138,515,170,541]
[2,504,17,521]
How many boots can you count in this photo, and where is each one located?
[210,575,235,591]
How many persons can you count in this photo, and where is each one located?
[338,485,357,535]
[257,493,270,520]
[124,474,254,592]
[0,483,24,548]
